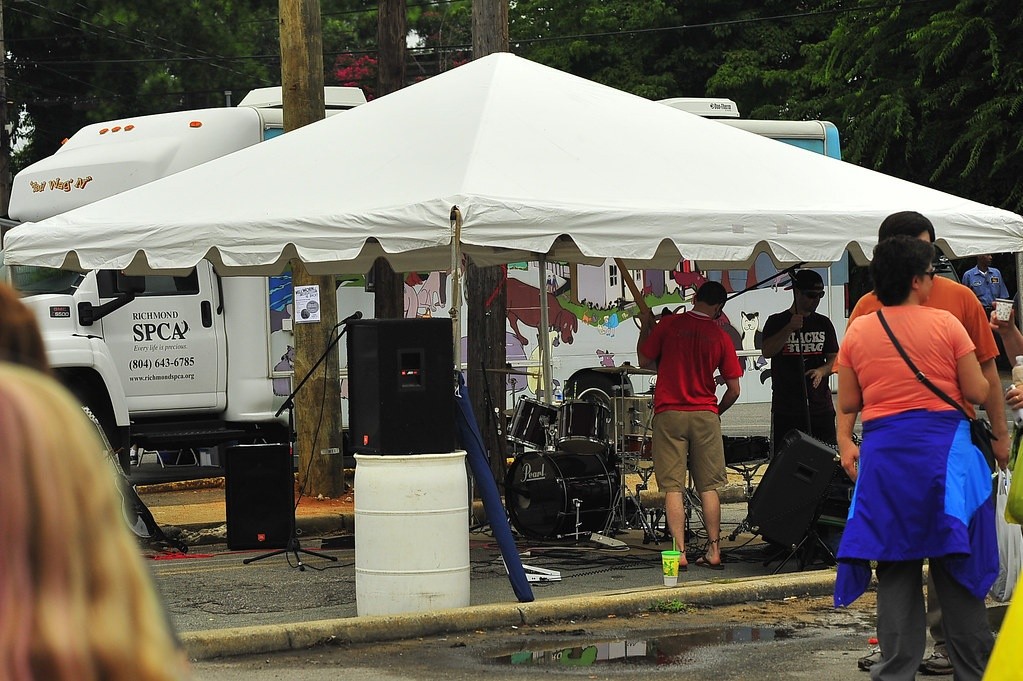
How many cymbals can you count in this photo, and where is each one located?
[502,408,516,417]
[619,410,643,415]
[592,366,658,376]
[474,368,542,376]
[633,389,655,395]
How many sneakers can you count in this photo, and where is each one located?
[858,645,882,671]
[919,652,954,674]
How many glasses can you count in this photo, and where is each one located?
[921,267,937,280]
[799,291,825,299]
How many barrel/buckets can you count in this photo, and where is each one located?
[353,449,471,617]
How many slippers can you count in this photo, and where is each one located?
[677,563,689,571]
[695,556,725,570]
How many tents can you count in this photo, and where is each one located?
[3,52,1023,415]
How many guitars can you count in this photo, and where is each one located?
[80,406,157,539]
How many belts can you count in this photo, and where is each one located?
[984,306,993,311]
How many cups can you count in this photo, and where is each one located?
[996,298,1014,321]
[661,550,681,586]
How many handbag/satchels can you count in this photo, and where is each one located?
[971,417,998,474]
[1004,420,1023,524]
[988,466,1022,602]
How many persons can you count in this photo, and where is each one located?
[637,280,743,571]
[0,287,196,681]
[987,300,1023,456]
[961,254,1009,370]
[832,211,1010,681]
[837,238,997,681]
[762,270,840,459]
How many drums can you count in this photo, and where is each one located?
[623,433,652,461]
[506,396,560,451]
[557,399,612,454]
[505,452,618,541]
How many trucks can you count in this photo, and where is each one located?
[0,86,849,475]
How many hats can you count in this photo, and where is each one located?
[784,269,824,290]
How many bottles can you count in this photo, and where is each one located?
[1012,355,1023,420]
[555,386,563,402]
